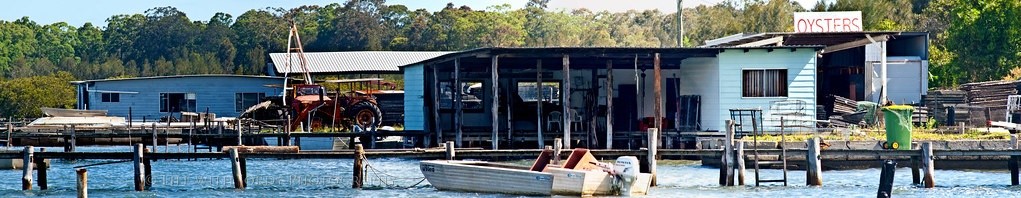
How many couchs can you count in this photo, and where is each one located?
[638,116,667,133]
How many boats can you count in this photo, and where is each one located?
[420,160,554,196]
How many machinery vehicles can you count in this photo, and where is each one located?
[291,83,382,130]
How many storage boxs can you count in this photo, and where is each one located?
[564,148,598,170]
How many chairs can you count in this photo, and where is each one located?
[570,109,584,133]
[548,111,562,132]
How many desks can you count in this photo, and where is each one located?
[729,109,764,136]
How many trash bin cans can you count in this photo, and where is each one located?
[882,104,915,149]
